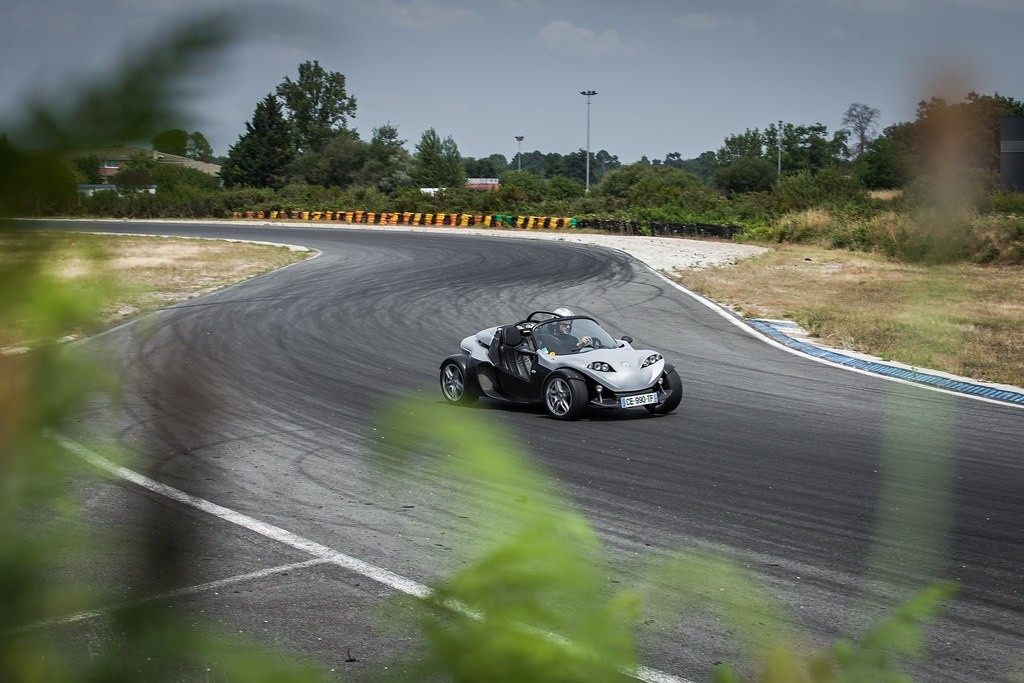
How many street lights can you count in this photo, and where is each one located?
[515,136,524,171]
[580,90,598,191]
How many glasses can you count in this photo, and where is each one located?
[561,323,571,327]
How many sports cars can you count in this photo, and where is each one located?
[437,308,683,423]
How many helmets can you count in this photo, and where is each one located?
[551,307,574,331]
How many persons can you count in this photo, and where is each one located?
[543,308,592,354]
